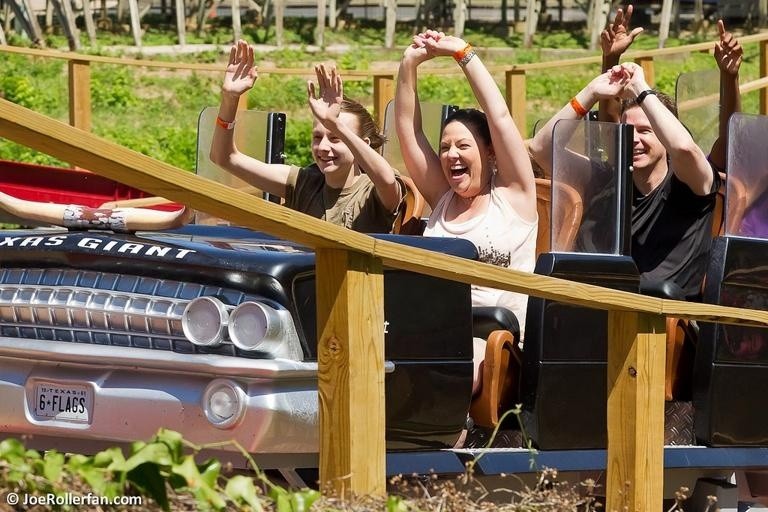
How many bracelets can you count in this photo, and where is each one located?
[571,96,590,118]
[215,117,237,130]
[451,43,476,68]
[636,89,656,107]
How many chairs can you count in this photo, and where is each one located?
[663,172,747,402]
[387,175,425,235]
[468,178,585,430]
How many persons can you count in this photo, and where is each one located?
[526,62,720,424]
[394,27,538,445]
[205,37,404,238]
[594,4,744,177]
